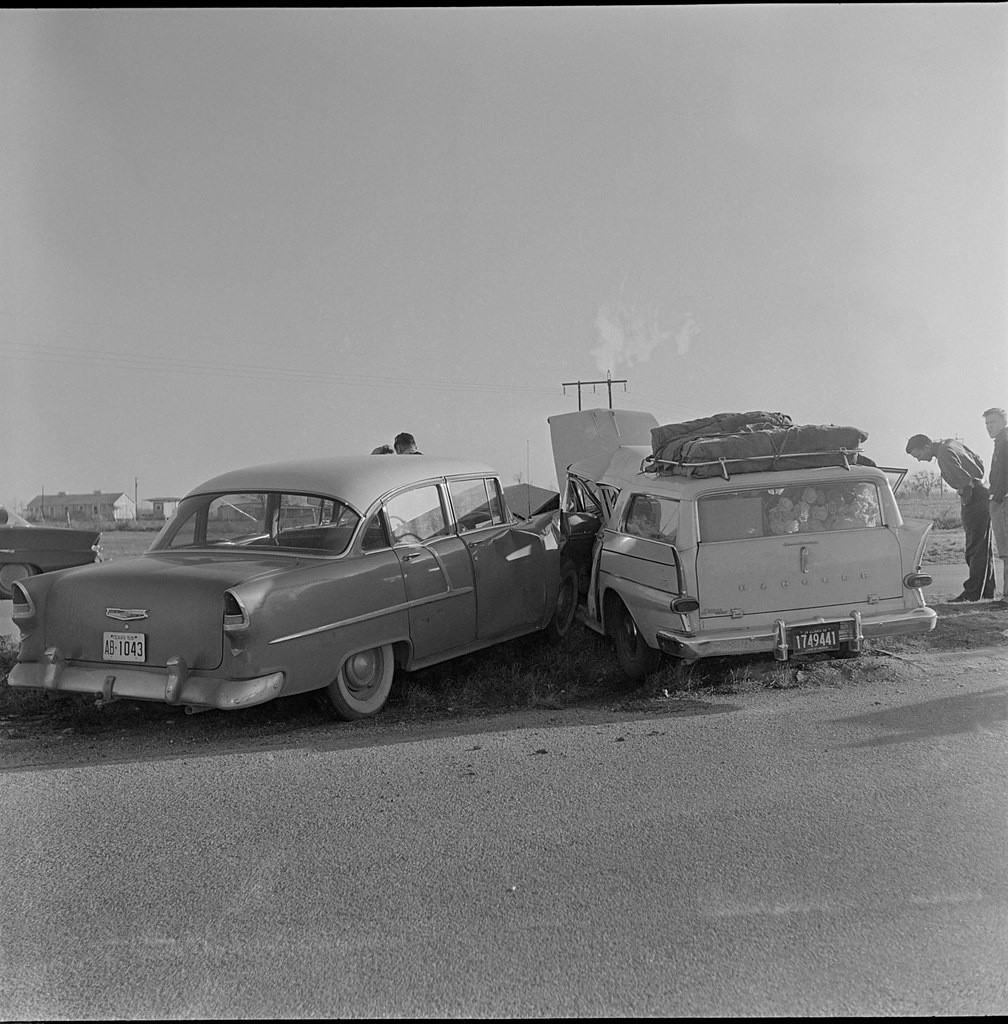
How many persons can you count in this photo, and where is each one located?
[983,408,1008,611]
[905,433,996,602]
[370,432,423,456]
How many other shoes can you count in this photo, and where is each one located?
[947,594,969,602]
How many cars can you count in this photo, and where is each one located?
[546,409,941,678]
[0,504,106,605]
[2,455,582,721]
